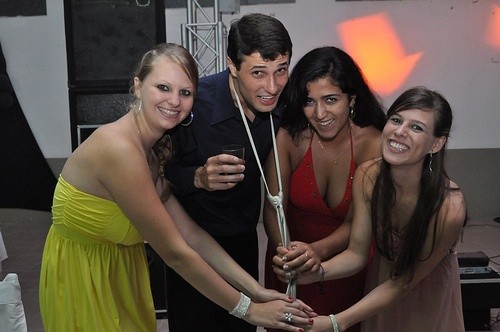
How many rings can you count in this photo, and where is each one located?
[285,313,293,322]
[305,252,310,259]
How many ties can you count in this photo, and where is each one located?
[232,82,296,299]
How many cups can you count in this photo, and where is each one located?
[221,144,245,175]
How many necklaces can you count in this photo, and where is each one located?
[315,124,354,166]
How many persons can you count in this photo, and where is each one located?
[263,46,386,332]
[164,13,293,332]
[273,87,467,332]
[39,43,318,332]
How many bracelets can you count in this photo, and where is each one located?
[229,292,251,318]
[319,264,324,281]
[329,314,339,332]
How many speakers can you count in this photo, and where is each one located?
[64,0,167,153]
